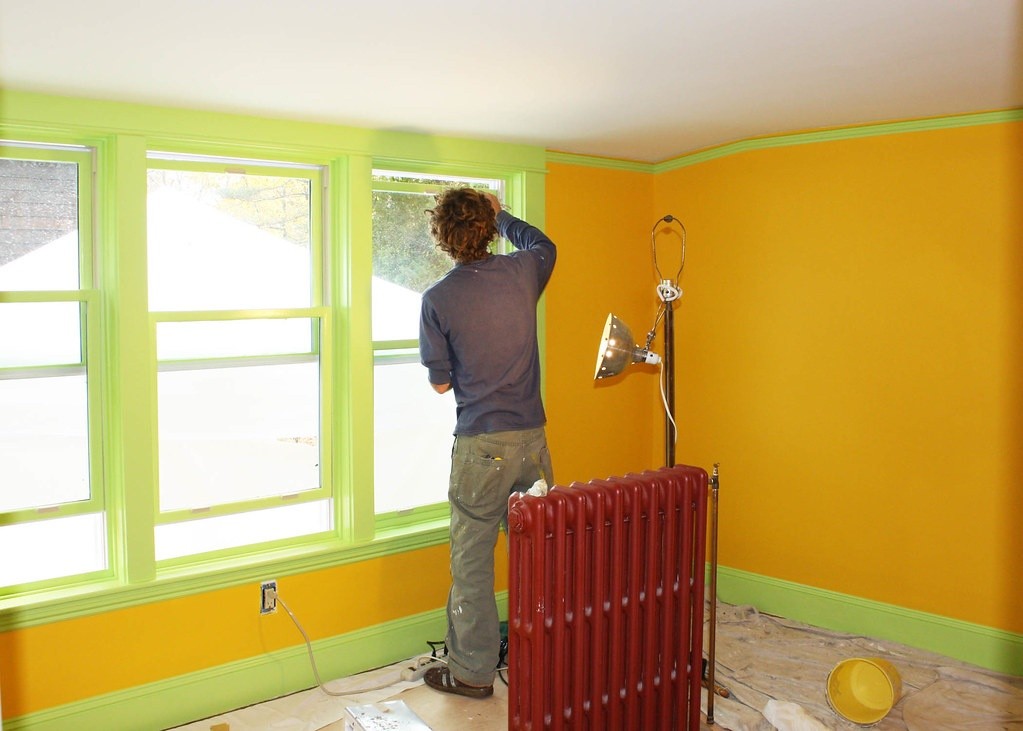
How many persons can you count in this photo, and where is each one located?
[419,188,556,698]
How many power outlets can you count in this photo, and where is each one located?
[261,581,278,616]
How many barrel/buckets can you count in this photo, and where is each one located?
[826,658,902,724]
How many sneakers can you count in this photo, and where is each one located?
[424,666,493,698]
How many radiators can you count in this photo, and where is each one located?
[505,463,719,731]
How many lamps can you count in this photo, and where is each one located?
[592,279,678,380]
